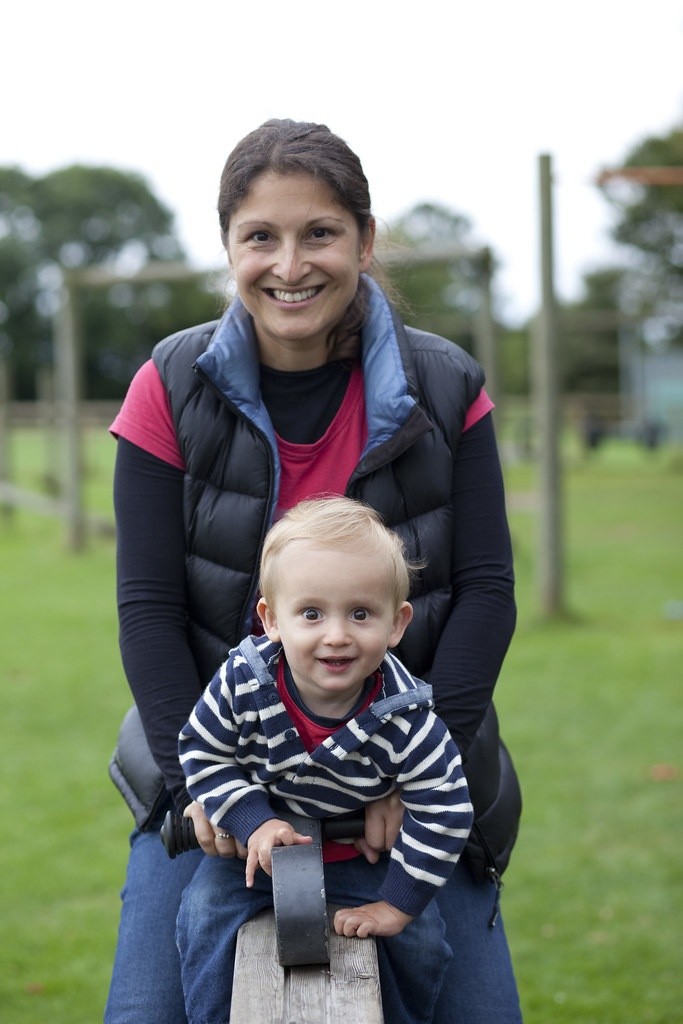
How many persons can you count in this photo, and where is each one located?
[106,118,525,1024]
